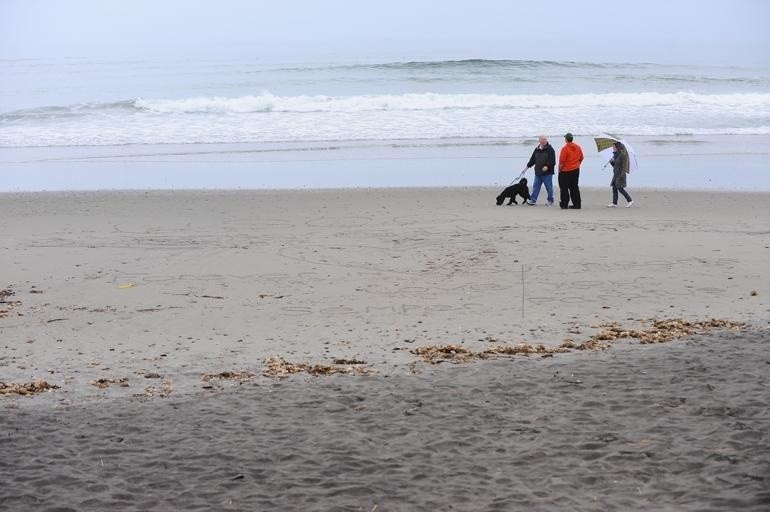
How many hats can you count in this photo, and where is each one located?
[564,133,573,138]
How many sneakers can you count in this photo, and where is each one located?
[560,202,580,208]
[546,200,553,206]
[626,201,634,208]
[527,200,536,205]
[607,204,617,208]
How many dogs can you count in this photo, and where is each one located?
[495,178,531,206]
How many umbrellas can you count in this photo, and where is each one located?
[594,132,638,175]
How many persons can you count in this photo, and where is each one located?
[607,142,634,208]
[525,134,555,206]
[557,133,584,209]
